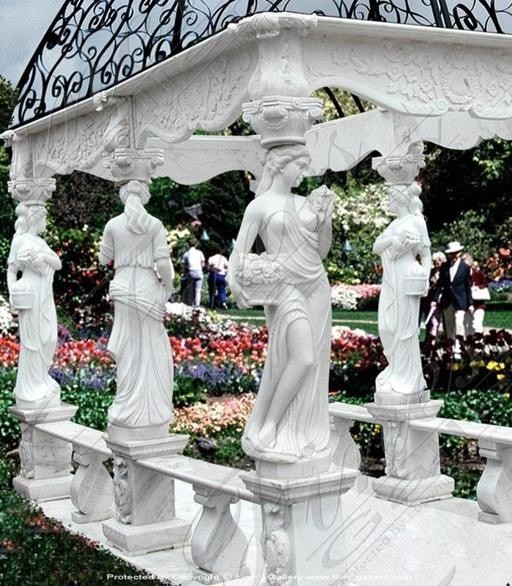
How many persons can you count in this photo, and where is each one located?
[374,186,431,395]
[182,238,206,307]
[420,241,492,340]
[207,242,230,310]
[6,203,62,409]
[228,144,336,465]
[97,179,174,429]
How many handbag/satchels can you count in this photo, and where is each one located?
[471,286,491,300]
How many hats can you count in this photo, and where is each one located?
[446,242,463,253]
